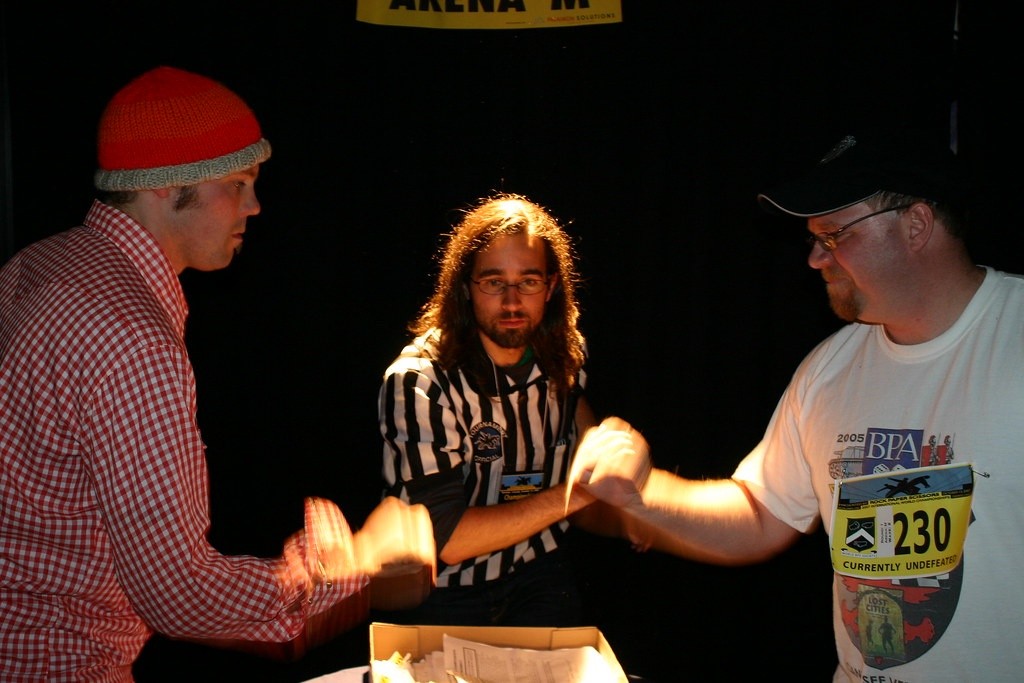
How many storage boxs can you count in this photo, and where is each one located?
[369,621,629,683]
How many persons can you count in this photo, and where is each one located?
[569,133,1024,683]
[0,66,437,683]
[378,195,599,626]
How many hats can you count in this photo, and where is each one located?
[754,111,960,216]
[93,66,273,192]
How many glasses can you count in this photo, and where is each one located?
[468,277,550,295]
[806,196,939,251]
